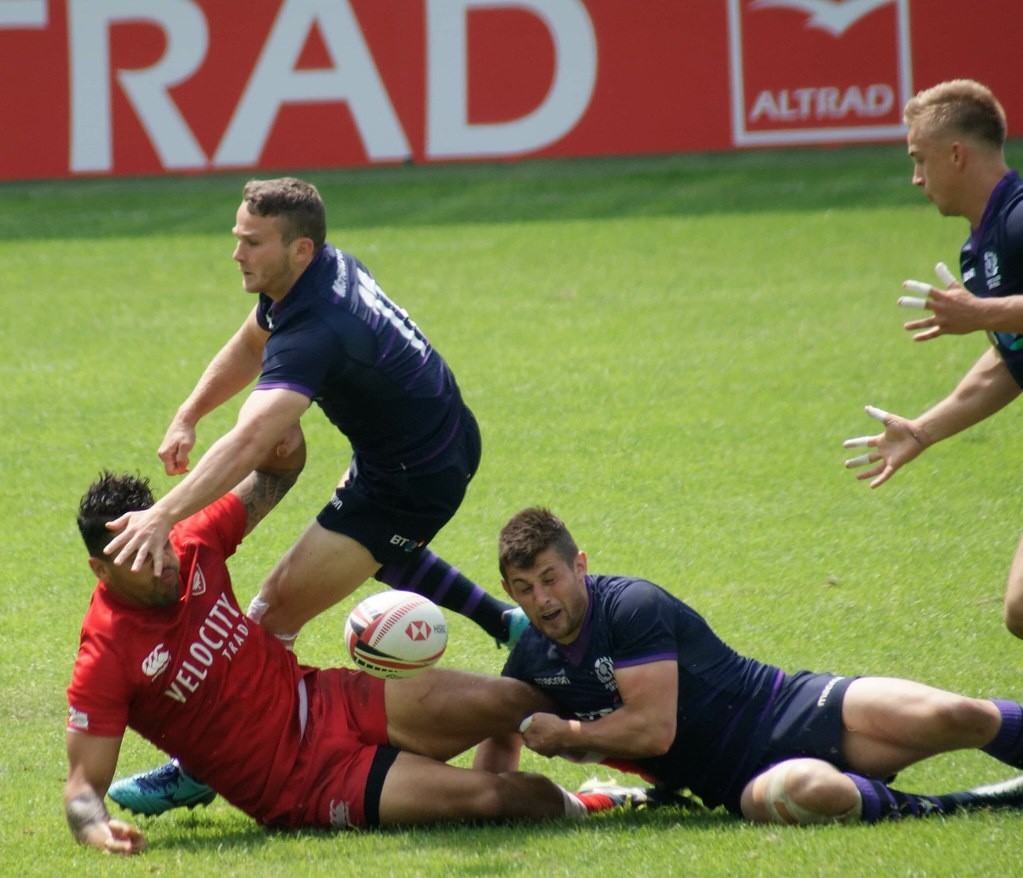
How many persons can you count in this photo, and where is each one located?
[106,178,530,820]
[844,79,1023,640]
[64,422,705,854]
[473,508,1023,828]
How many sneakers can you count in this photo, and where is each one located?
[107,758,217,817]
[974,776,1023,804]
[578,777,703,811]
[496,606,532,653]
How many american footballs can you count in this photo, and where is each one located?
[345,590,449,678]
